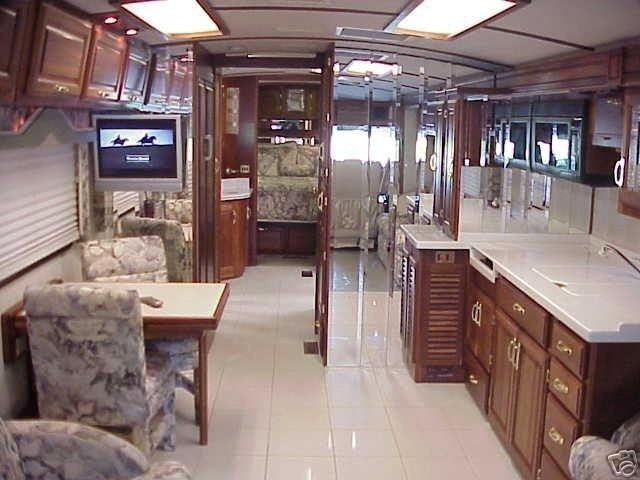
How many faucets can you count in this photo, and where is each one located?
[598,243,640,275]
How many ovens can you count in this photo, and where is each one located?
[530,98,624,184]
[507,102,531,170]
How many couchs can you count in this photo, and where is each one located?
[330,158,402,252]
[255,141,320,225]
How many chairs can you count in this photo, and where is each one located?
[1,419,191,479]
[144,199,194,225]
[23,285,177,457]
[121,215,194,282]
[71,235,201,426]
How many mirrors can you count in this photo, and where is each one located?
[89,113,194,274]
[325,37,496,365]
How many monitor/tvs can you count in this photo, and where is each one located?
[91,113,185,193]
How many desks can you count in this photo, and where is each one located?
[1,281,230,445]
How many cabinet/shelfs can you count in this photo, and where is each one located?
[119,37,191,113]
[432,85,512,239]
[481,94,623,187]
[1,0,128,109]
[614,86,640,218]
[398,224,470,381]
[459,247,640,480]
